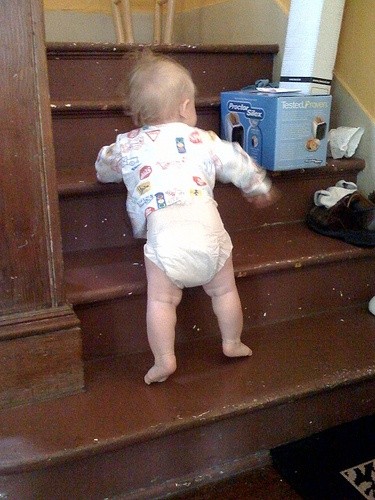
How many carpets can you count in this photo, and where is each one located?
[269,416,375,500]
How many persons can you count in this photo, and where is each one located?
[94,48,272,386]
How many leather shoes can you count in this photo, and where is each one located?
[305,190,375,248]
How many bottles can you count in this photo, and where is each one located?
[246,117,262,166]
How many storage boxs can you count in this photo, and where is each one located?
[219,90,333,171]
